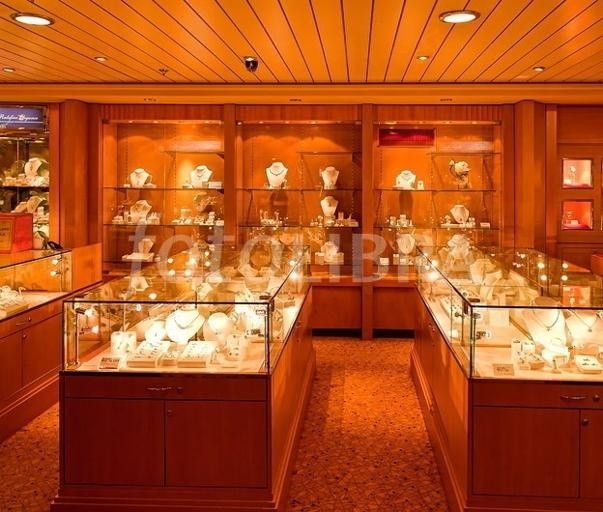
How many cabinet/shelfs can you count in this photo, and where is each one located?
[48,243,317,512]
[0,106,52,249]
[0,242,103,441]
[409,244,603,512]
[93,119,228,272]
[229,117,367,342]
[369,119,505,339]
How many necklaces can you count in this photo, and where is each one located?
[173,311,199,330]
[24,159,45,175]
[265,167,285,179]
[399,175,412,181]
[451,240,467,249]
[530,301,562,332]
[573,308,600,333]
[193,170,208,180]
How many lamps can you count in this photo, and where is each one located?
[438,10,480,24]
[9,12,54,27]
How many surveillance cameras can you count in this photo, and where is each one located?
[244,57,258,72]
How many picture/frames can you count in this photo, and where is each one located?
[561,157,595,189]
[561,198,595,231]
